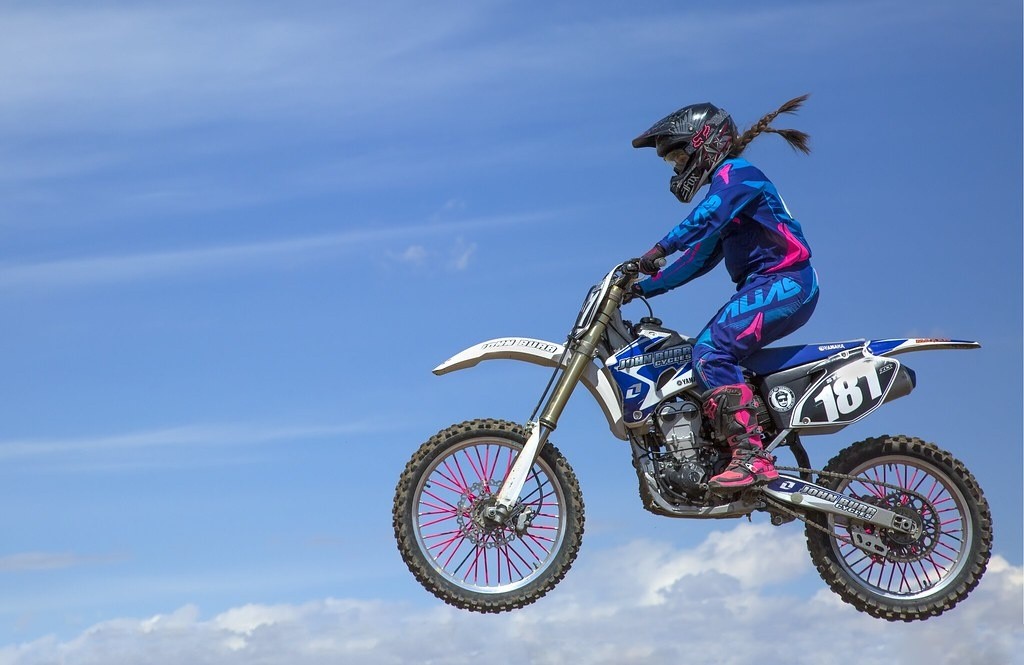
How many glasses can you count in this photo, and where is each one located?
[664,149,689,175]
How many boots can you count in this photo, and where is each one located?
[701,383,780,487]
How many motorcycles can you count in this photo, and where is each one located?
[391,258,994,624]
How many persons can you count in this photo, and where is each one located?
[622,92,820,495]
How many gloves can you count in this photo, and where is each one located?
[639,243,668,276]
[622,283,643,305]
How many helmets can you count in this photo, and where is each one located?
[631,102,738,203]
